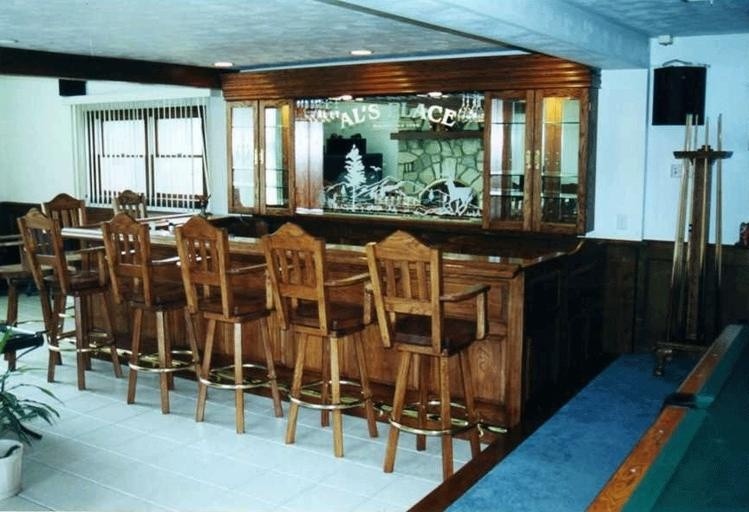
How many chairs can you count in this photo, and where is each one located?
[263,221,378,458]
[366,230,491,482]
[0,234,76,370]
[100,213,208,414]
[41,193,86,228]
[113,189,147,221]
[16,208,123,390]
[174,215,283,434]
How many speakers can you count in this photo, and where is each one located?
[652,66,707,125]
[60,79,86,95]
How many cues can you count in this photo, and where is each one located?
[665,115,722,345]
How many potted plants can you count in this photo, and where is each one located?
[0,322,66,502]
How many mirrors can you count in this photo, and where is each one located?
[291,57,485,219]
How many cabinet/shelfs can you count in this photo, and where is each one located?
[221,66,293,218]
[485,52,602,235]
